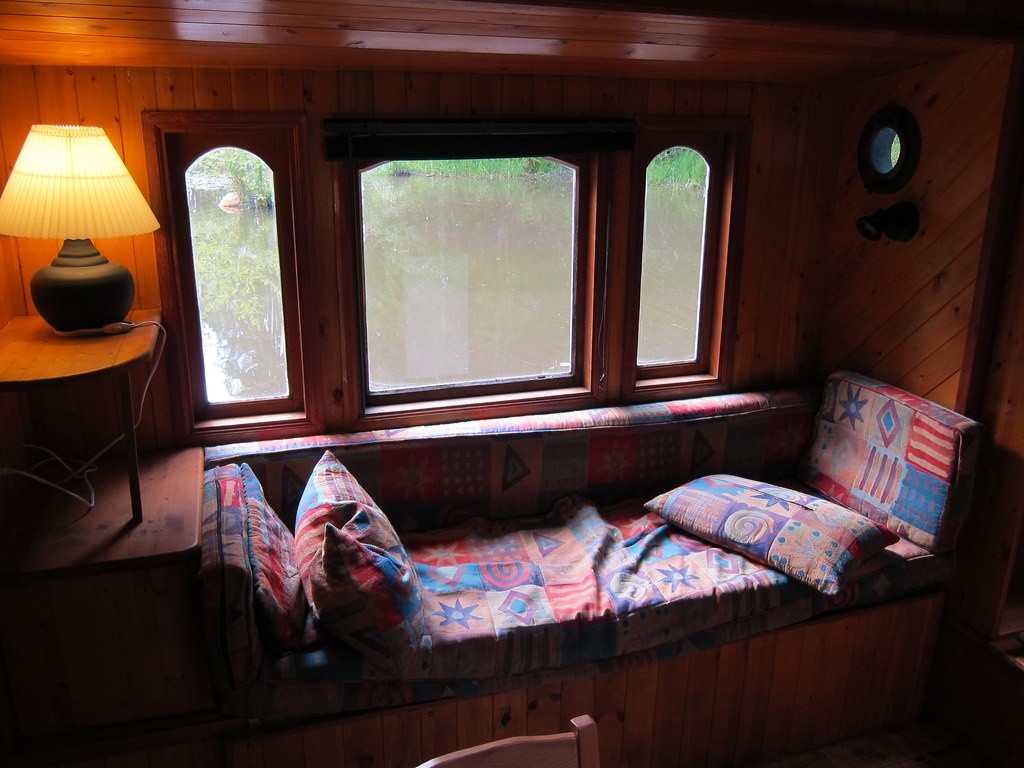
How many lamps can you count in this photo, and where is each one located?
[1,124,168,338]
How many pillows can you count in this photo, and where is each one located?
[292,442,430,689]
[237,461,311,676]
[639,473,904,599]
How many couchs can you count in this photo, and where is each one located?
[198,369,981,726]
